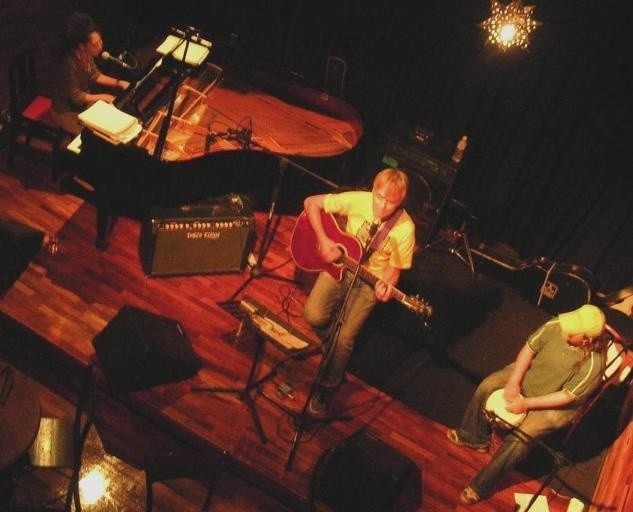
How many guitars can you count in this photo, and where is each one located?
[289,207,434,319]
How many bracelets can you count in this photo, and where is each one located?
[116,79,120,89]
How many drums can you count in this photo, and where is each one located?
[481,387,536,460]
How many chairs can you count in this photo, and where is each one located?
[6,47,79,182]
[553,324,628,452]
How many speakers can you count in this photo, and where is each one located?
[91,303,203,394]
[311,428,422,512]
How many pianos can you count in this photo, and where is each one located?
[67,25,365,250]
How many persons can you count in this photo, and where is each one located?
[304,170,416,416]
[447,305,607,506]
[21,28,132,126]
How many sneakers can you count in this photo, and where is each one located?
[446,428,489,453]
[309,391,328,414]
[461,487,479,505]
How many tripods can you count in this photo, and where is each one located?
[258,247,368,469]
[228,160,305,302]
[188,337,277,444]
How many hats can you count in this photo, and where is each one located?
[558,303,606,338]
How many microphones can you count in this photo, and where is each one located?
[366,217,381,247]
[102,51,128,69]
